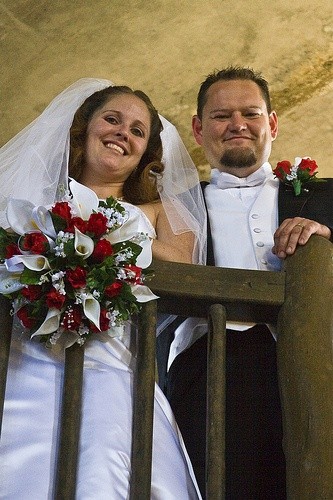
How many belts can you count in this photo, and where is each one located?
[227,327,271,338]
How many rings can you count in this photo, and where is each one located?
[295,224,305,228]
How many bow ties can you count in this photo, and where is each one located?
[217,167,266,189]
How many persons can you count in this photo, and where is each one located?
[163,65,332,500]
[0,77,208,500]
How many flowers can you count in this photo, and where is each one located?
[0,176,160,351]
[272,156,328,196]
